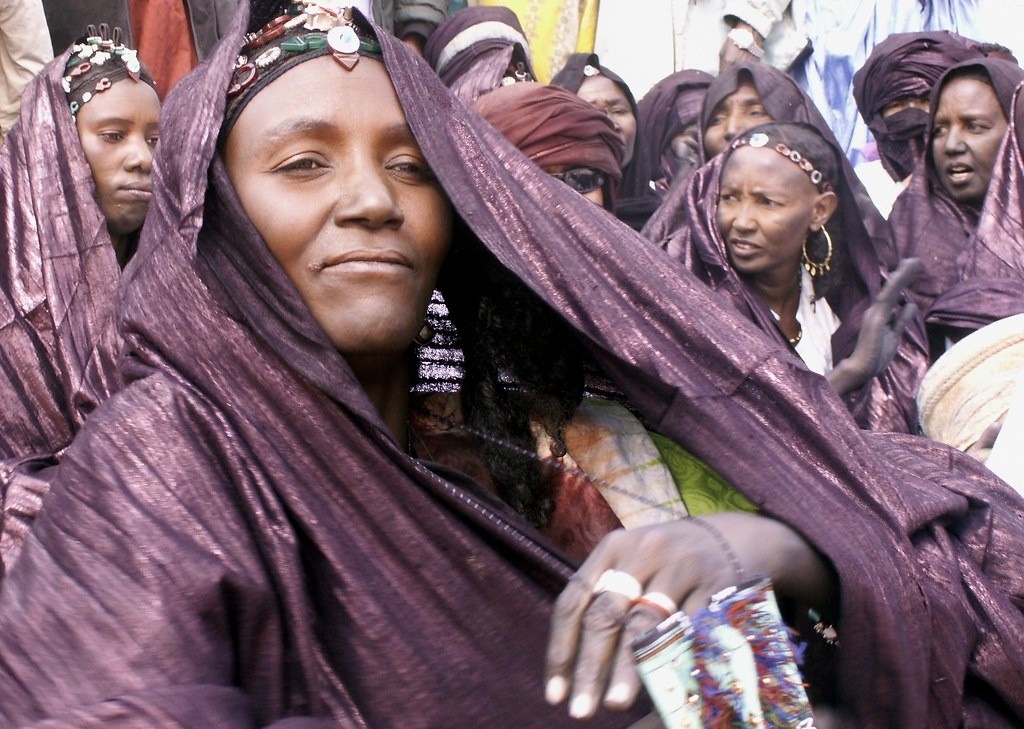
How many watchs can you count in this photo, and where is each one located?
[727,28,766,60]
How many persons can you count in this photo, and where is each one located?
[591,1,811,106]
[885,57,1024,321]
[637,119,929,436]
[788,0,982,171]
[851,29,1019,221]
[0,23,164,582]
[695,58,889,267]
[548,51,663,234]
[914,311,1024,467]
[922,78,1024,370]
[613,69,717,201]
[469,81,626,216]
[247,0,451,61]
[1,1,602,158]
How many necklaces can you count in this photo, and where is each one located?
[405,418,435,464]
[788,268,802,344]
[396,397,817,728]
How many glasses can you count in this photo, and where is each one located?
[548,166,605,195]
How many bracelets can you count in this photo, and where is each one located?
[806,605,842,650]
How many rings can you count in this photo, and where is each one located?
[630,592,677,619]
[589,567,643,603]
